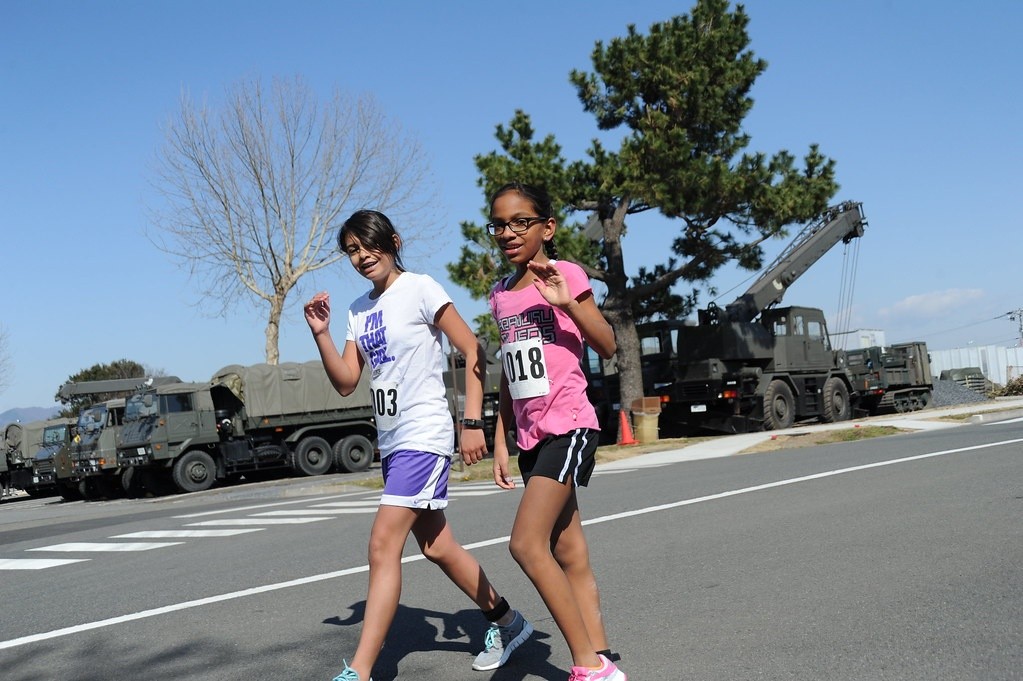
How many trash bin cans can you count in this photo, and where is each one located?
[631,396,662,443]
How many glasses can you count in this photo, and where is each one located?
[486,217,548,236]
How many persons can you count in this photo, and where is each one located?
[304,211,534,681]
[487,183,626,681]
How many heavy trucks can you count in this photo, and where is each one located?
[0,358,379,501]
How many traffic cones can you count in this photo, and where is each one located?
[616,411,640,445]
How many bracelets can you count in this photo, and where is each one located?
[463,419,484,428]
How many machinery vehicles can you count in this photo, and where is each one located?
[671,199,869,430]
[846,341,933,413]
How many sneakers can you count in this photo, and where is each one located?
[568,654,627,681]
[472,610,534,670]
[332,659,373,681]
